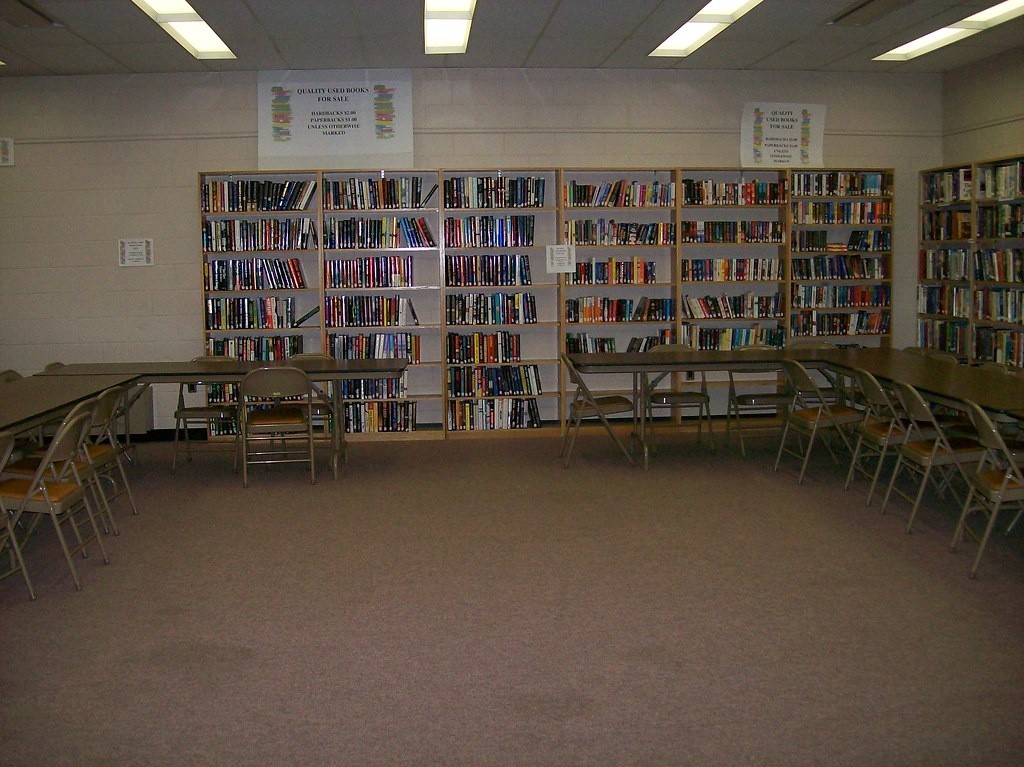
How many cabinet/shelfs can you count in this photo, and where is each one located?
[912,154,1024,447]
[199,169,898,439]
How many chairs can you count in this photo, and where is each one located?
[559,343,1024,579]
[1,352,341,601]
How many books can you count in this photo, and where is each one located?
[565,296,674,323]
[446,292,538,325]
[443,176,545,209]
[445,254,532,286]
[564,218,676,245]
[444,215,535,248]
[443,331,546,432]
[918,161,1024,417]
[681,221,787,244]
[681,291,786,350]
[681,179,788,205]
[322,178,436,433]
[201,180,316,211]
[681,258,785,281]
[566,329,676,355]
[791,173,893,349]
[201,217,316,437]
[565,257,656,284]
[563,180,677,208]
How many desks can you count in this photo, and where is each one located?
[0,373,142,529]
[35,358,408,480]
[825,345,1024,424]
[569,350,854,454]
